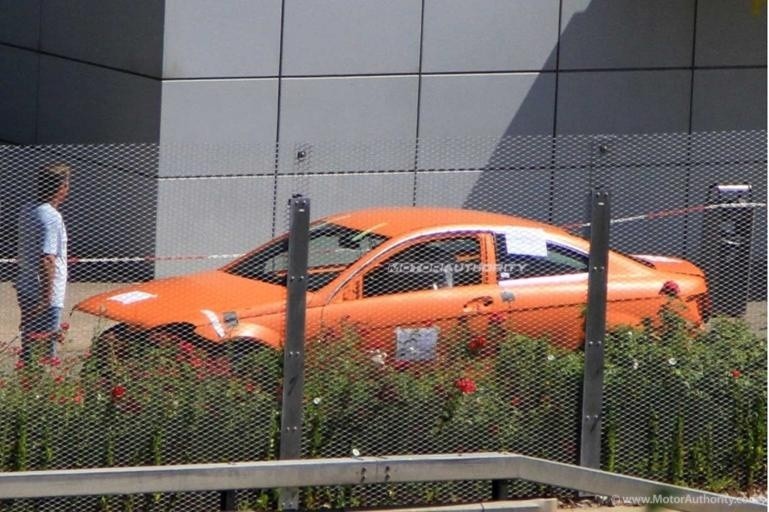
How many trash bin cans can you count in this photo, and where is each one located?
[702,184,752,317]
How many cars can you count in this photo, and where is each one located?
[69,205,714,384]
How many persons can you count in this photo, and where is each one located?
[16,162,68,367]
